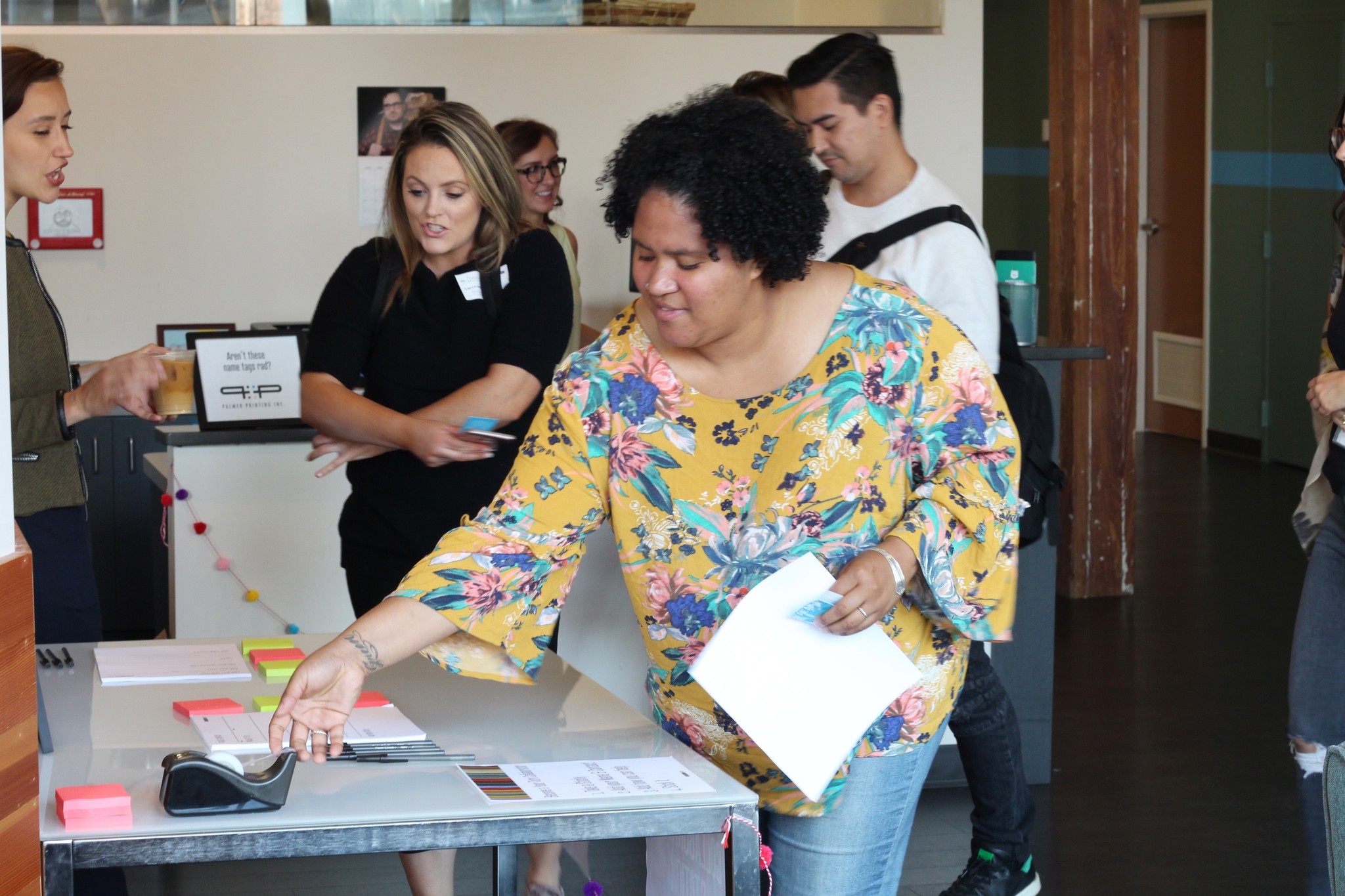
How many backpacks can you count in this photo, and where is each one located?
[834,211,1062,552]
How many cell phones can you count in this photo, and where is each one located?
[458,430,517,448]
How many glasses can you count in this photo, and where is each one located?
[514,158,567,184]
[383,102,403,111]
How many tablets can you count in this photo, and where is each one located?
[184,330,310,430]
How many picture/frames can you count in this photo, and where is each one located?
[186,330,308,432]
[26,188,104,250]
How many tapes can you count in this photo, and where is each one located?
[204,751,244,776]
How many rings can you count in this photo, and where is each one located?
[311,730,328,737]
[857,607,867,620]
[1340,413,1345,420]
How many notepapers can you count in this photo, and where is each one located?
[55,783,133,829]
[249,648,306,665]
[258,659,304,677]
[253,696,282,712]
[173,698,244,718]
[241,638,294,655]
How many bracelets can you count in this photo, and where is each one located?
[863,547,906,595]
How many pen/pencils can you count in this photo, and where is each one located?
[46,649,64,668]
[36,649,51,668]
[62,647,74,666]
[326,740,475,763]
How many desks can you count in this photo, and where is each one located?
[39,637,763,896]
[143,425,351,639]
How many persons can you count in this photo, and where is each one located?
[784,31,1043,896]
[359,90,413,156]
[268,83,1022,896]
[1,43,173,644]
[301,99,574,893]
[1282,108,1345,896]
[728,70,815,164]
[493,119,603,368]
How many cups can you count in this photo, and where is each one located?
[151,350,196,415]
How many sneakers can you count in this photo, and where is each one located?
[936,848,1040,896]
